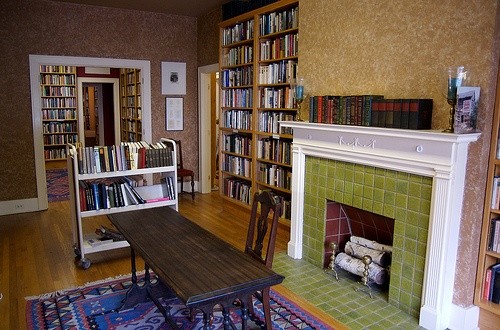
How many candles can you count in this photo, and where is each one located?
[297,86,303,100]
[447,78,460,96]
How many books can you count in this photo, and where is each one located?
[79,176,176,212]
[309,95,433,130]
[483,127,500,305]
[88,225,125,246]
[77,141,175,175]
[120,67,142,142]
[39,65,82,160]
[221,7,300,221]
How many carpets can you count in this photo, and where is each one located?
[46,168,70,201]
[25,268,355,330]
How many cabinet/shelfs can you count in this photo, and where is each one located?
[40,64,80,162]
[119,68,143,143]
[219,0,299,228]
[82,84,100,138]
[473,67,500,316]
[66,138,178,269]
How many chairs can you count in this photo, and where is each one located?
[170,139,195,200]
[187,191,281,330]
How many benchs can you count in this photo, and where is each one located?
[105,214,285,330]
